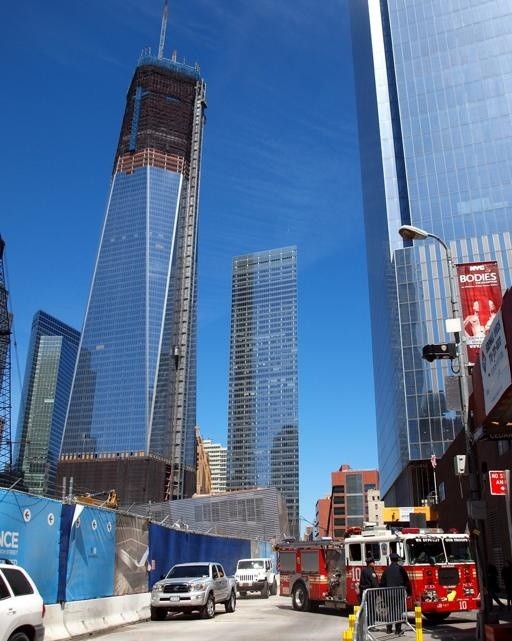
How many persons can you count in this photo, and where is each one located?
[358,558,380,632]
[463,300,499,336]
[380,553,413,638]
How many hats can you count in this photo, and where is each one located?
[391,554,398,560]
[365,557,374,562]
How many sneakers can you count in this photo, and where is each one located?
[388,630,392,633]
[396,630,404,634]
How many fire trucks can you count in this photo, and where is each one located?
[274,528,481,621]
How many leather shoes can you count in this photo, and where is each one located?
[371,628,380,631]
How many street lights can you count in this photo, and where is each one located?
[397,225,499,641]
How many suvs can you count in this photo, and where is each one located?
[150,563,236,619]
[233,558,277,598]
[0,557,46,641]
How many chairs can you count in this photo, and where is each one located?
[391,539,451,561]
